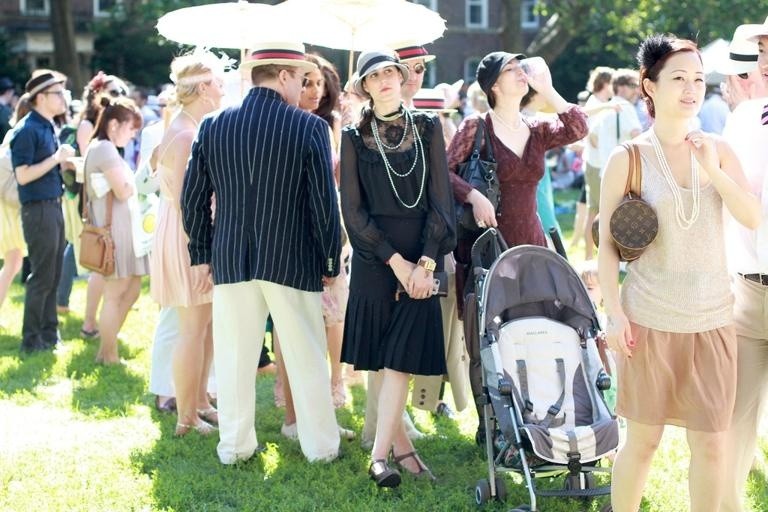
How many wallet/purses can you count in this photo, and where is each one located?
[397,272,448,297]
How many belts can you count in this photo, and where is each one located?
[24,197,62,204]
[739,272,768,286]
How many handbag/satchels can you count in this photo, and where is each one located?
[455,161,501,234]
[592,192,658,262]
[79,224,115,275]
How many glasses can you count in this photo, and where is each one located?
[108,86,126,97]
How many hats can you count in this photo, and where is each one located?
[715,16,767,76]
[26,74,66,101]
[238,27,532,113]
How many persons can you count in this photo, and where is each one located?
[0,18,768,510]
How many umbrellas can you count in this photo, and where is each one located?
[273,0,448,92]
[155,1,305,80]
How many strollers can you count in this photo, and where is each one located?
[467,226,621,512]
